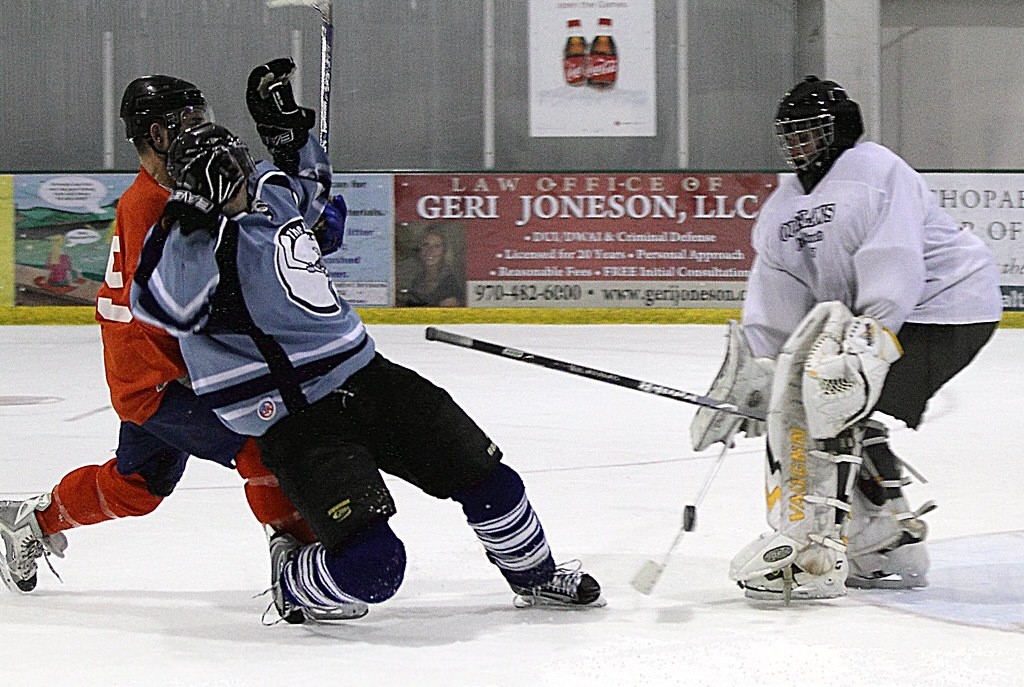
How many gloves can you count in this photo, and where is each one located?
[174,150,244,211]
[310,195,347,256]
[247,55,318,179]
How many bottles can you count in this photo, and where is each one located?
[587,17,617,90]
[562,19,590,88]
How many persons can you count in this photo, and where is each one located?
[409,225,460,307]
[0,55,609,629]
[720,73,1005,609]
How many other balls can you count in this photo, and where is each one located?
[684,506,697,532]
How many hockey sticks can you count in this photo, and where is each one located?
[424,324,766,421]
[265,0,332,154]
[631,418,746,596]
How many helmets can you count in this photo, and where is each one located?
[119,74,207,141]
[165,121,257,200]
[774,75,865,196]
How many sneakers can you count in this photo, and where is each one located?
[251,533,312,626]
[514,559,608,609]
[0,492,68,595]
[845,498,938,589]
[309,597,369,618]
[742,524,848,599]
